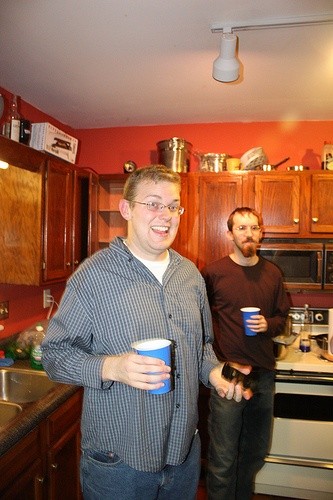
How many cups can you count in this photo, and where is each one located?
[130,337,173,395]
[240,307,260,336]
[225,158,240,171]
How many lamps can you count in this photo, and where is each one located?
[212,26,241,82]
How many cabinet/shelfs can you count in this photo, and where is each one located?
[0,388,83,500]
[0,145,131,287]
[178,170,333,269]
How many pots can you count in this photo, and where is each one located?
[256,157,290,170]
[198,153,229,171]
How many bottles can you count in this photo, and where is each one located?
[5,93,20,142]
[320,141,333,170]
[30,324,45,370]
[299,304,311,351]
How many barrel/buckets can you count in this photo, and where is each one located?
[156,136,193,173]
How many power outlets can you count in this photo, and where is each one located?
[43,289,51,309]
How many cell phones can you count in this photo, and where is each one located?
[222,364,257,391]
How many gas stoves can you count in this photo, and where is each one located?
[274,307,333,383]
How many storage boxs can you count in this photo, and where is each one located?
[28,123,79,164]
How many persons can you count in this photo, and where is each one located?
[44,165,253,500]
[198,208,291,500]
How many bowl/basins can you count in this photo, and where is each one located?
[316,333,329,349]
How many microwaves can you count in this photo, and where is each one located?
[256,238,333,290]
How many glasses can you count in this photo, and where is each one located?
[131,200,186,217]
[233,225,262,232]
